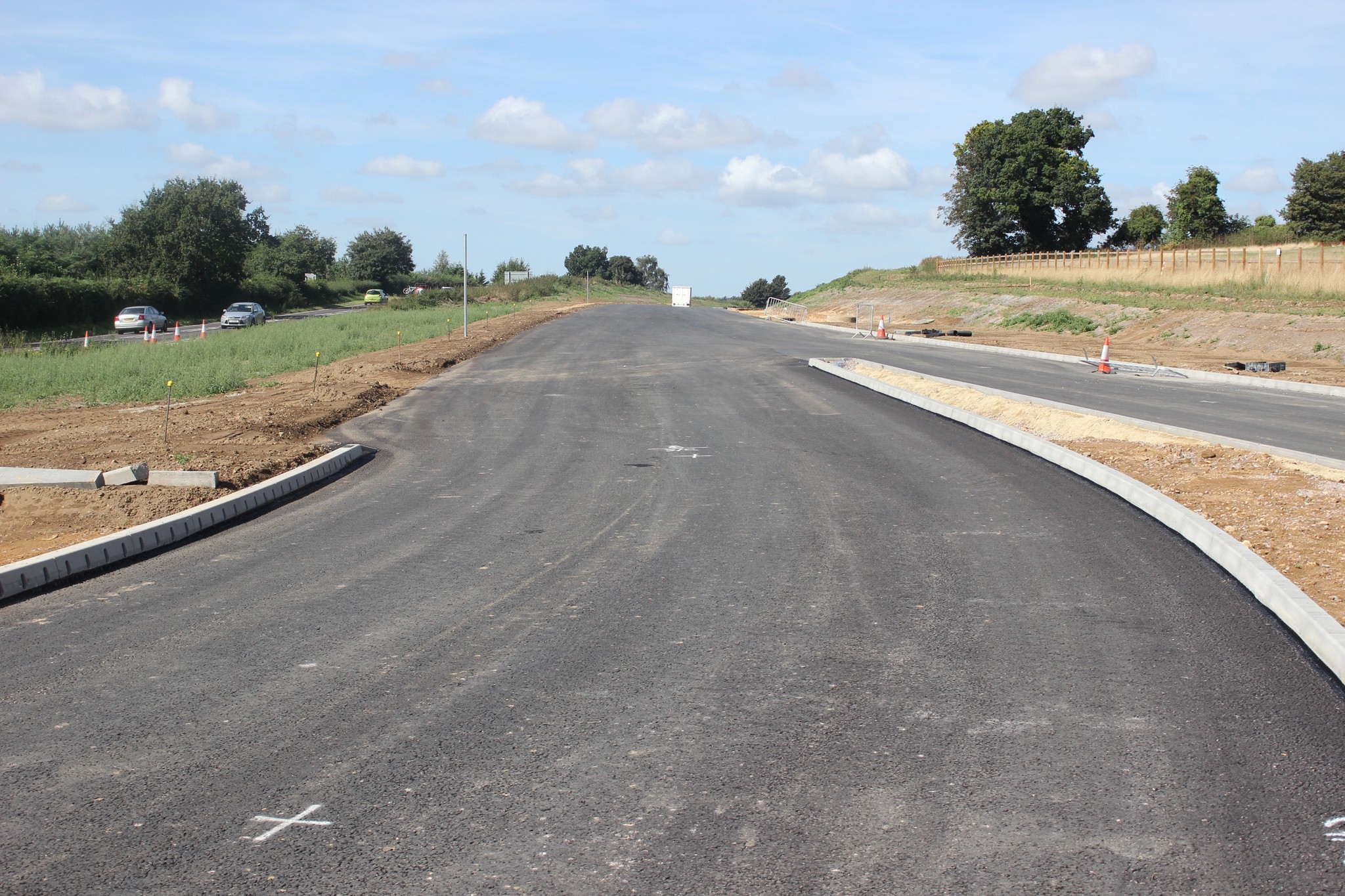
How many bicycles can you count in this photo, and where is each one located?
[403,285,418,294]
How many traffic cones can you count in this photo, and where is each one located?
[875,314,889,339]
[143,325,150,343]
[199,318,207,338]
[174,322,181,341]
[84,330,90,348]
[1094,337,1113,373]
[150,324,158,344]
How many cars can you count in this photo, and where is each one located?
[114,306,168,334]
[440,287,455,292]
[364,289,389,305]
[221,303,266,330]
